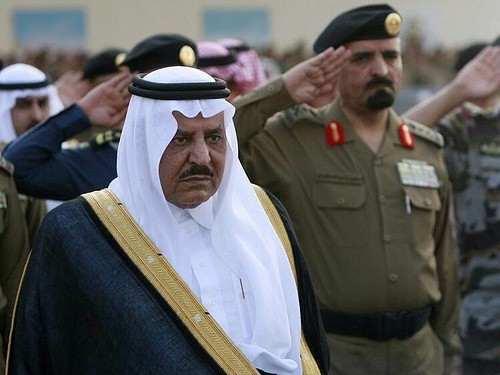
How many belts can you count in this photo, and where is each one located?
[319,307,436,341]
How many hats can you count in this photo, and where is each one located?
[313,3,403,54]
[117,33,196,71]
[79,47,126,79]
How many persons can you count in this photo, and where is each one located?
[9,66,331,375]
[0,4,500,375]
